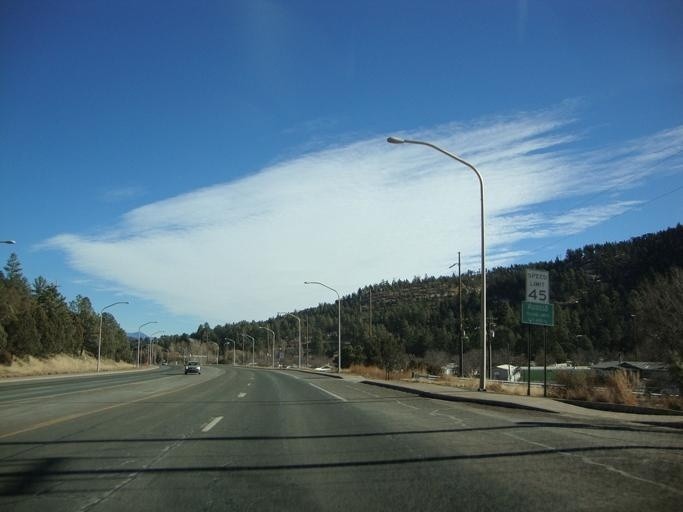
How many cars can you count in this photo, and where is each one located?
[184,361,201,374]
[162,361,168,366]
[315,365,333,370]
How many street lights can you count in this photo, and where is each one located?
[97,301,129,372]
[304,281,341,373]
[212,327,274,368]
[449,252,464,377]
[138,321,165,368]
[0,240,16,245]
[386,136,486,391]
[277,311,301,368]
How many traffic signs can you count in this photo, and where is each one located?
[527,269,550,304]
